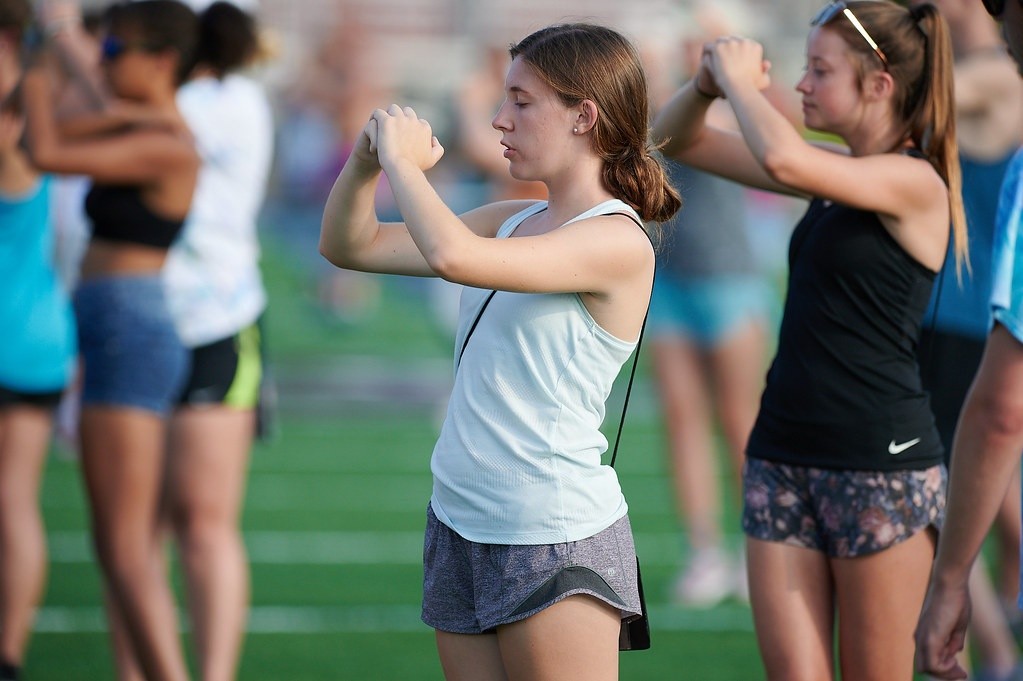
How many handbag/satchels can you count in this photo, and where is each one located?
[617,557,651,650]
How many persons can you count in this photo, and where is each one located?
[0,1,97,681]
[294,3,681,680]
[911,0,1023,681]
[174,0,276,680]
[650,0,972,681]
[21,0,200,681]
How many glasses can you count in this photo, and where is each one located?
[102,36,158,59]
[810,0,889,70]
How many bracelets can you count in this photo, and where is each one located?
[694,73,719,100]
